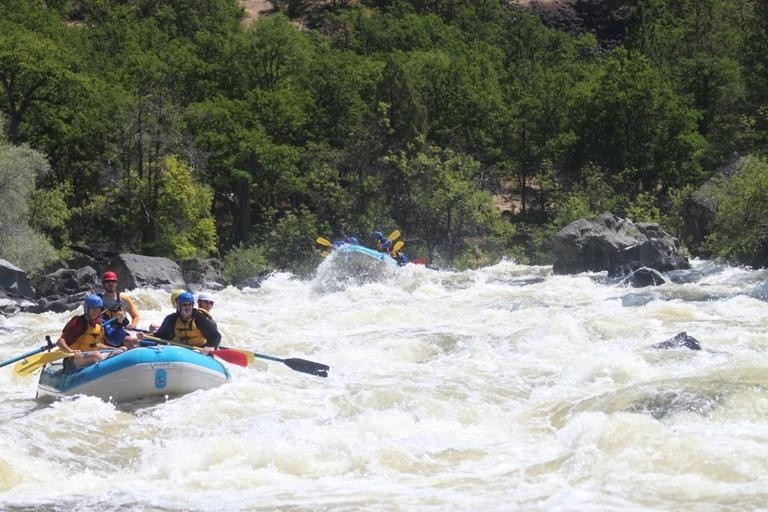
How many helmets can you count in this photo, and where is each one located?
[102,271,117,289]
[332,230,408,266]
[170,290,214,311]
[83,293,103,319]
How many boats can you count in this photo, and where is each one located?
[315,244,400,277]
[35,329,232,403]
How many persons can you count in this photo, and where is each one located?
[328,231,406,265]
[57,271,222,373]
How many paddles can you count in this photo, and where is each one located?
[16,331,256,377]
[132,327,329,376]
[317,230,404,259]
